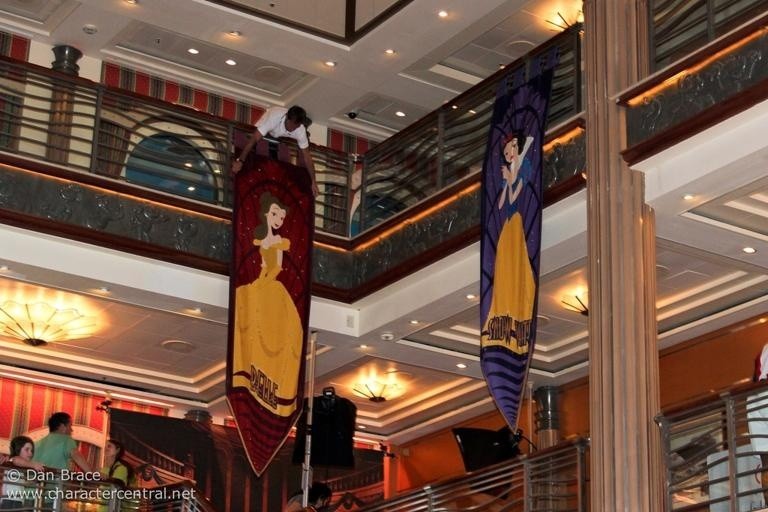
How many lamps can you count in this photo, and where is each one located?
[559,291,589,318]
[350,380,407,402]
[0,300,103,346]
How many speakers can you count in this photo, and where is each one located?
[291,394,357,470]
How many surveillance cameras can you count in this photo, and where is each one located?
[346,108,360,119]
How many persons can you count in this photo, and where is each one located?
[480,129,537,355]
[233,192,304,417]
[286,483,332,512]
[0,412,139,512]
[231,106,319,197]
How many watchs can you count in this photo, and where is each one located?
[236,158,245,165]
